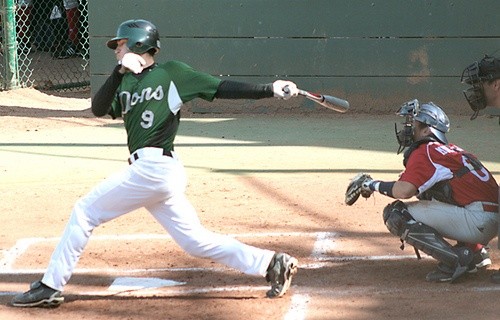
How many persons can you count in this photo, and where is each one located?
[346,100,500,282]
[14,0,81,59]
[11,20,300,306]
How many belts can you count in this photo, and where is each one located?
[482,204,500,212]
[128,147,173,166]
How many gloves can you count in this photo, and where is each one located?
[273,80,298,101]
[119,52,146,74]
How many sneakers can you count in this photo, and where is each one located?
[266,252,298,299]
[425,246,474,284]
[457,243,491,273]
[11,280,65,308]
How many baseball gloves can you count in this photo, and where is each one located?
[345,175,371,206]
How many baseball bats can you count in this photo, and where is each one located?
[284,87,349,113]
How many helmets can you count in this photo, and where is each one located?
[394,99,450,155]
[106,19,160,54]
